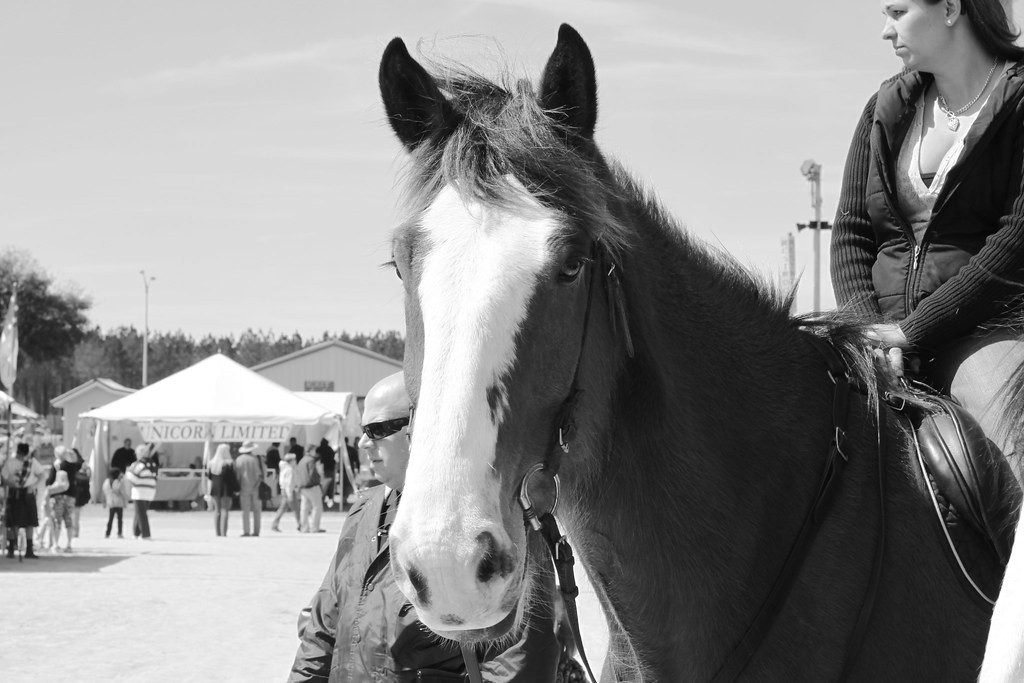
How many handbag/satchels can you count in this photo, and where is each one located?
[259,481,271,500]
[47,463,70,495]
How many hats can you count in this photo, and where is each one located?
[238,441,258,453]
[55,444,77,463]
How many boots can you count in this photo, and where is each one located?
[6,540,14,558]
[24,539,38,559]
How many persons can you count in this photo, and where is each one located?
[829,1,1024,496]
[294,444,327,533]
[265,442,281,494]
[289,437,304,464]
[102,470,128,538]
[234,440,267,537]
[73,462,91,537]
[44,419,85,553]
[1,443,45,559]
[126,444,159,542]
[207,444,241,538]
[271,453,302,532]
[316,437,336,509]
[287,371,559,683]
[111,439,136,478]
[336,437,360,505]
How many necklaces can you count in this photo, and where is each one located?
[941,56,999,133]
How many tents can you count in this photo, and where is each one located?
[291,392,363,493]
[77,354,344,512]
[0,391,40,466]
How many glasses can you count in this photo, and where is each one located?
[359,416,410,440]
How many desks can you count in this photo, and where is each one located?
[156,476,207,510]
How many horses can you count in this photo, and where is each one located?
[376,22,1024,683]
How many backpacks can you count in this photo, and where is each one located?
[74,463,91,506]
